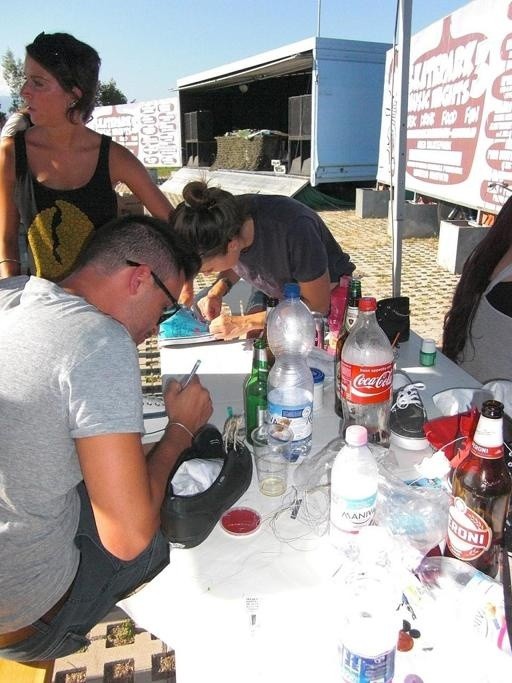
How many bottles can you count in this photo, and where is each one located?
[244,336,274,446]
[302,402,511,679]
[265,281,316,462]
[327,275,395,447]
[418,338,440,366]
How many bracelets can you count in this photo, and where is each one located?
[212,276,233,296]
[164,422,197,440]
[0,259,20,266]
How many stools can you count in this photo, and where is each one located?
[0,652,55,683]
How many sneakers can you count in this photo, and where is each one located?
[384,369,431,451]
[159,424,253,549]
[157,301,234,347]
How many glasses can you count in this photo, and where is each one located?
[125,259,182,326]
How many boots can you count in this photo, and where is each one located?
[372,296,411,345]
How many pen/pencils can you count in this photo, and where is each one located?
[181,360,201,390]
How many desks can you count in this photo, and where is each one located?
[158,309,512,683]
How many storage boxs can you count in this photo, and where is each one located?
[115,184,145,221]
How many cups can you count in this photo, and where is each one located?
[251,426,294,498]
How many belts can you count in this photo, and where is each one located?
[0,579,75,650]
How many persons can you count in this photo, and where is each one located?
[2,105,35,137]
[168,180,357,340]
[0,215,215,664]
[441,196,512,386]
[0,32,193,309]
